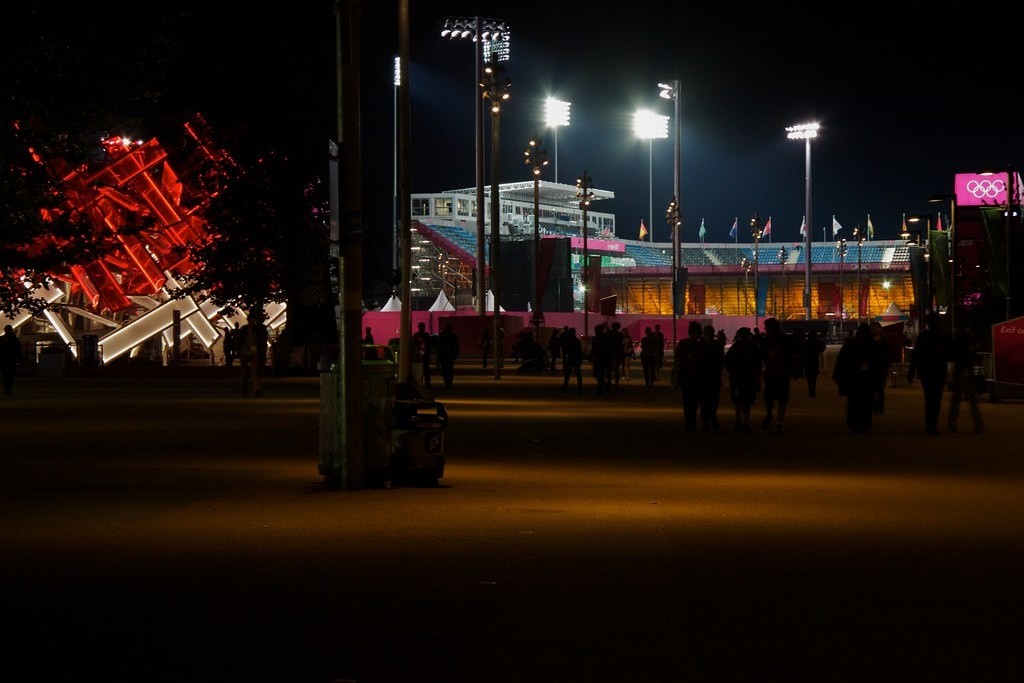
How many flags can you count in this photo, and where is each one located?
[833,219,842,234]
[937,217,941,230]
[762,220,771,238]
[800,221,806,237]
[729,221,736,237]
[699,223,706,237]
[639,224,648,238]
[868,220,873,237]
[602,224,609,238]
[903,218,907,231]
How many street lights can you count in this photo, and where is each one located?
[575,170,596,333]
[907,216,937,312]
[656,77,683,311]
[750,215,764,327]
[835,236,849,318]
[544,95,572,183]
[440,16,510,366]
[479,51,513,382]
[852,223,869,328]
[633,108,670,241]
[927,194,957,335]
[523,134,550,310]
[785,121,822,320]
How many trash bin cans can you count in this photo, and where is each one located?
[316,343,399,487]
[391,400,447,487]
[974,351,993,379]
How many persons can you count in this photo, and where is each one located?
[552,322,665,391]
[499,328,506,367]
[0,325,21,396]
[365,327,373,343]
[675,318,827,432]
[411,322,460,388]
[224,322,240,365]
[482,327,493,366]
[832,321,894,433]
[905,314,986,433]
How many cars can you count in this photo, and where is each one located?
[389,338,400,353]
[336,343,398,376]
